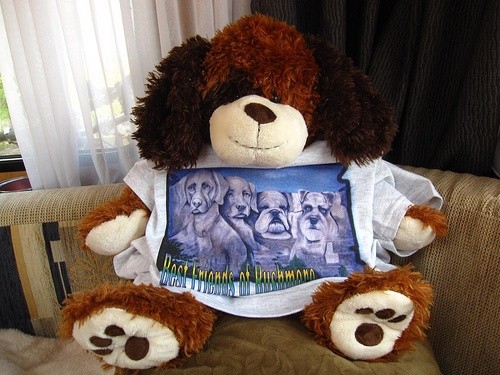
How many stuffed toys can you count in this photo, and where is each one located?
[57,14,449,375]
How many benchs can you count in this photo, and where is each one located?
[1,155,498,374]
